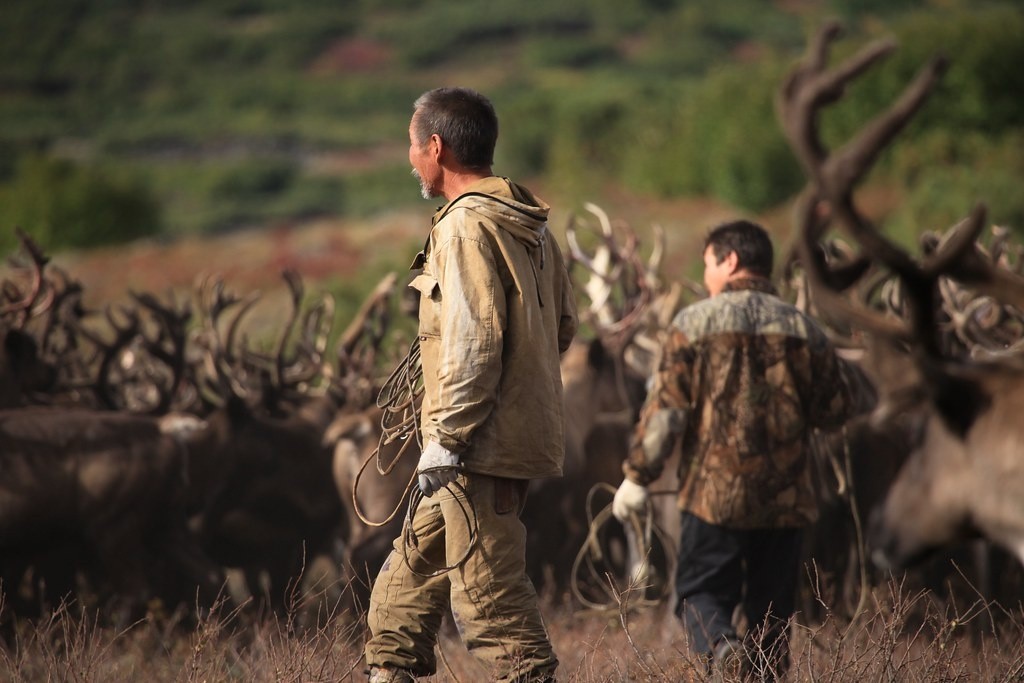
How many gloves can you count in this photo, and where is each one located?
[417,442,463,498]
[613,479,649,523]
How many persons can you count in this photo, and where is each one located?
[611,221,858,683]
[365,87,578,683]
